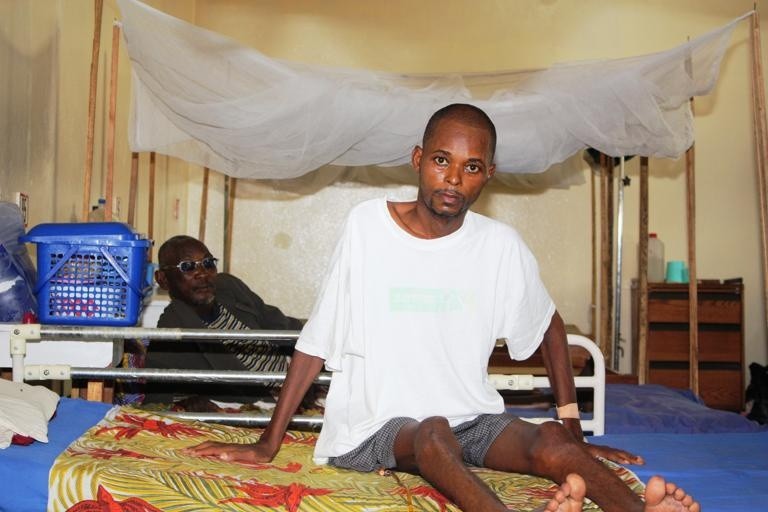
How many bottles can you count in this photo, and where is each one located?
[647,232,665,284]
[87,197,107,223]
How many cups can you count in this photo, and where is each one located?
[664,260,686,285]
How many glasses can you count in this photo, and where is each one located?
[159,257,218,274]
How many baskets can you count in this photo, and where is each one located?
[31,239,151,327]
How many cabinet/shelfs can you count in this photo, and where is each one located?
[629,278,748,413]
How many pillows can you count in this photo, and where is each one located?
[0,380,60,449]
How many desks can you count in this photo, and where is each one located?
[0,322,125,403]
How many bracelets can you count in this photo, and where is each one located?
[555,402,580,420]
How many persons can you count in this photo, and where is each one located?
[183,103,703,512]
[142,236,329,413]
